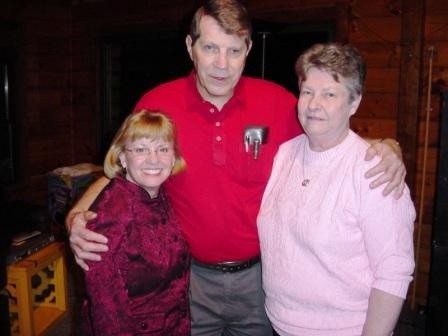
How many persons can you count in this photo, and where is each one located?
[255,41,417,336]
[65,0,409,336]
[79,107,193,336]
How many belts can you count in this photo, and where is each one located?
[191,255,262,273]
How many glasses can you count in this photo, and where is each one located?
[124,147,173,156]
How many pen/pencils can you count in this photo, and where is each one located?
[254,140,259,161]
[244,136,250,153]
